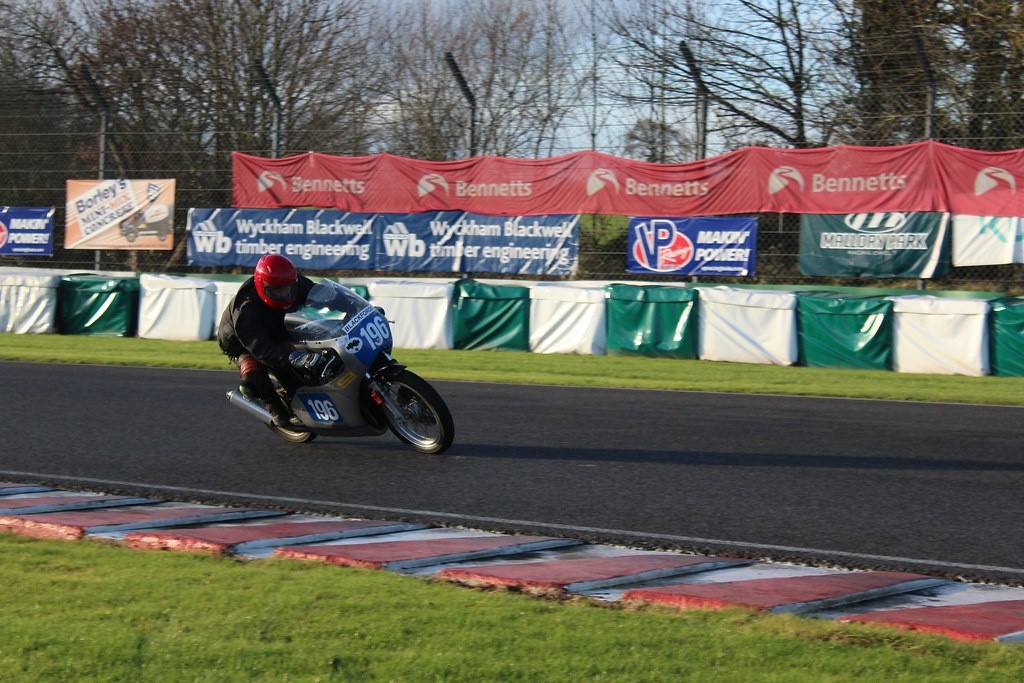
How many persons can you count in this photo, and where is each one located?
[215,252,366,428]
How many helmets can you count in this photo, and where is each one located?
[254,253,297,310]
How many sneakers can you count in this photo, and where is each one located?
[265,395,289,428]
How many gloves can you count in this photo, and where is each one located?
[288,351,328,371]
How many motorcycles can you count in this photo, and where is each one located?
[222,277,456,455]
